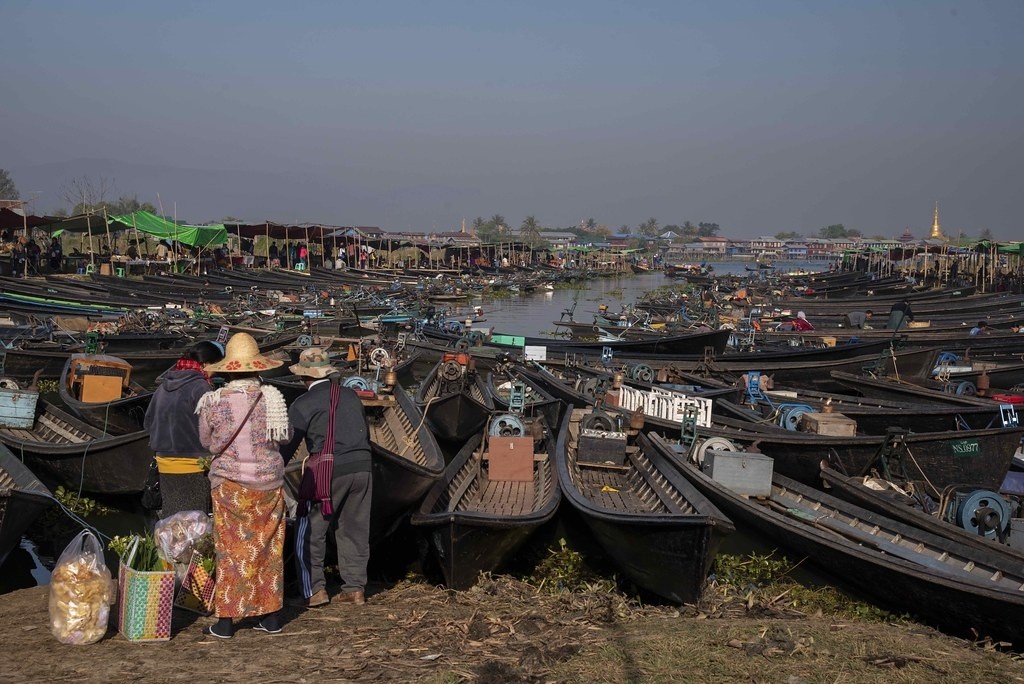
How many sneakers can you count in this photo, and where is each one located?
[330,591,365,605]
[288,589,329,607]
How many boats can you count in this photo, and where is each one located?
[555,372,740,605]
[410,377,564,599]
[284,345,449,547]
[0,257,1024,539]
[0,373,155,499]
[0,442,53,568]
[647,403,1024,623]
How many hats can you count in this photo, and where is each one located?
[288,348,339,378]
[203,332,284,372]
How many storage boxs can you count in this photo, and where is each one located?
[803,412,857,437]
[0,388,39,430]
[817,336,837,347]
[79,375,122,403]
[577,428,627,466]
[703,450,774,497]
[488,437,534,482]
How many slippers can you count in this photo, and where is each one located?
[252,621,282,633]
[202,626,231,638]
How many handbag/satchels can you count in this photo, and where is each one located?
[174,550,217,616]
[154,510,213,564]
[47,529,112,645]
[296,454,334,518]
[118,534,176,643]
[140,460,162,510]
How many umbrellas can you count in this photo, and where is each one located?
[659,231,679,243]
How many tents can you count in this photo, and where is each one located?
[234,221,432,270]
[433,240,546,268]
[605,234,659,245]
[548,246,645,267]
[0,200,234,276]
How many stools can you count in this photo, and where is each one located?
[76,267,85,274]
[85,264,97,274]
[115,268,125,277]
[295,262,305,271]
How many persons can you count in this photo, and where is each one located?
[196,333,295,638]
[278,347,372,606]
[0,232,231,272]
[241,238,377,267]
[144,341,226,520]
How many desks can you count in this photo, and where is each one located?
[63,256,254,276]
